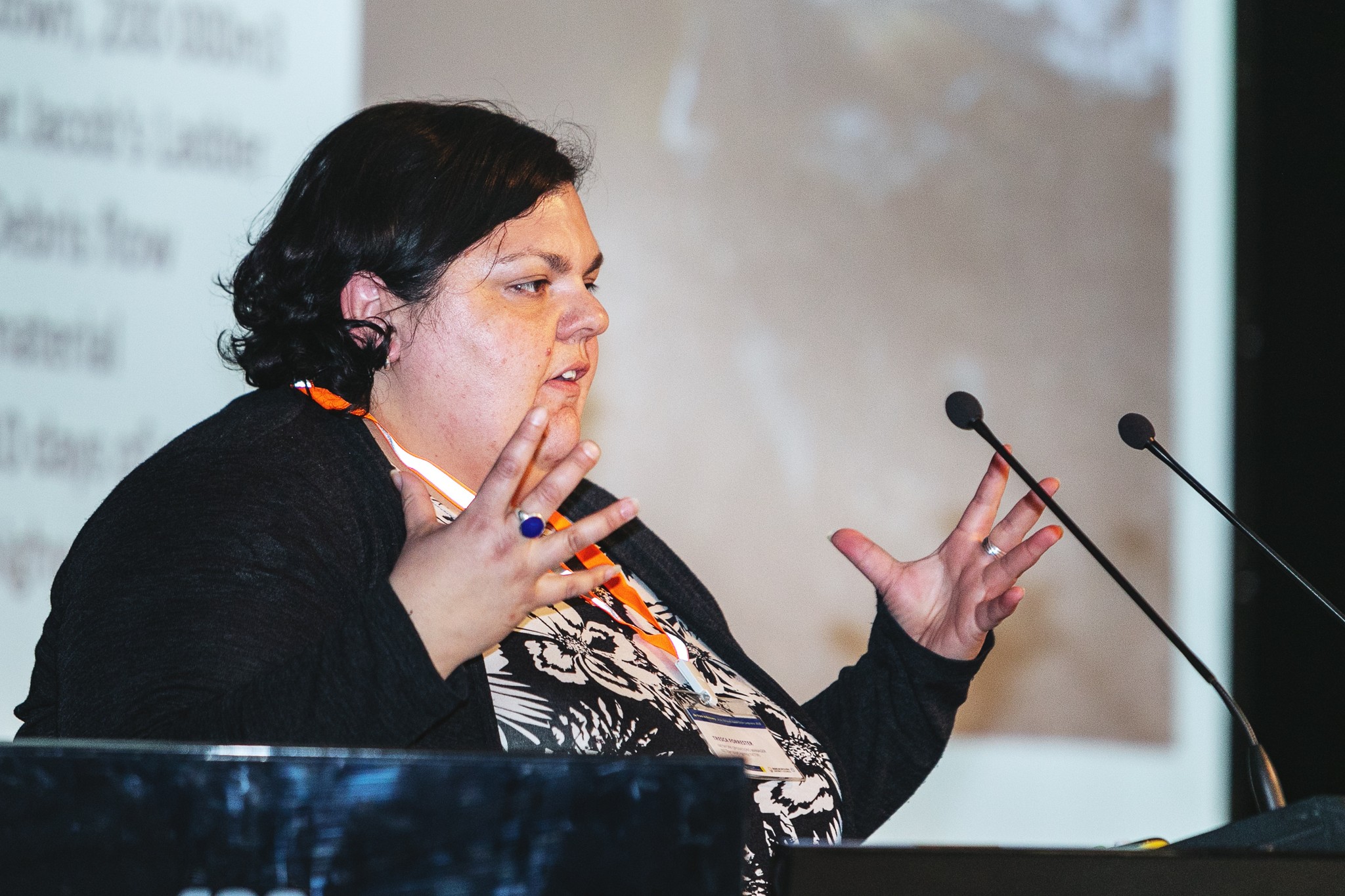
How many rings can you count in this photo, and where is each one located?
[982,536,1006,560]
[513,505,547,539]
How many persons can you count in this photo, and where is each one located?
[0,98,1063,896]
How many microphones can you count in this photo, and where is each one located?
[943,391,1345,862]
[1117,413,1344,621]
